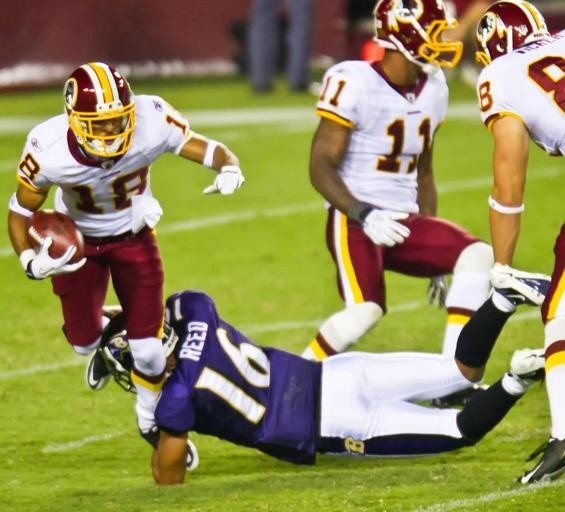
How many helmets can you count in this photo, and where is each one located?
[475,1,551,66]
[99,310,179,395]
[371,0,463,71]
[61,61,134,169]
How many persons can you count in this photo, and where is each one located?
[469,0,564,488]
[249,0,309,92]
[299,0,495,360]
[83,261,557,485]
[7,61,246,469]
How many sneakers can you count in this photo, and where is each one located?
[87,305,119,389]
[517,437,564,486]
[136,421,198,472]
[511,347,546,383]
[492,265,552,305]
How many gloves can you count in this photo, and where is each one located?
[204,166,245,197]
[426,275,445,306]
[360,205,410,246]
[27,238,86,281]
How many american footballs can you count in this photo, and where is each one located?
[29,210,86,265]
